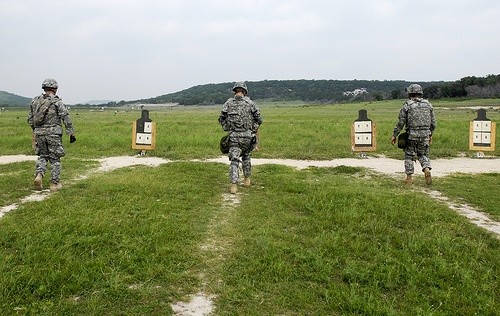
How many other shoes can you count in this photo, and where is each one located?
[231,184,238,193]
[50,182,62,191]
[34,175,42,191]
[244,177,250,187]
[404,175,412,185]
[424,168,432,186]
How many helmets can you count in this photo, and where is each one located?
[407,84,423,95]
[232,80,248,93]
[42,78,58,89]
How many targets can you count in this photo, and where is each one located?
[350,108,377,152]
[469,108,496,152]
[131,108,157,150]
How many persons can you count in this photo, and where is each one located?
[391,84,436,185]
[27,78,76,191]
[217,81,263,194]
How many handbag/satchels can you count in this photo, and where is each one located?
[220,134,230,154]
[398,132,410,149]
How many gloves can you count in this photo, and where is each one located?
[69,135,76,143]
